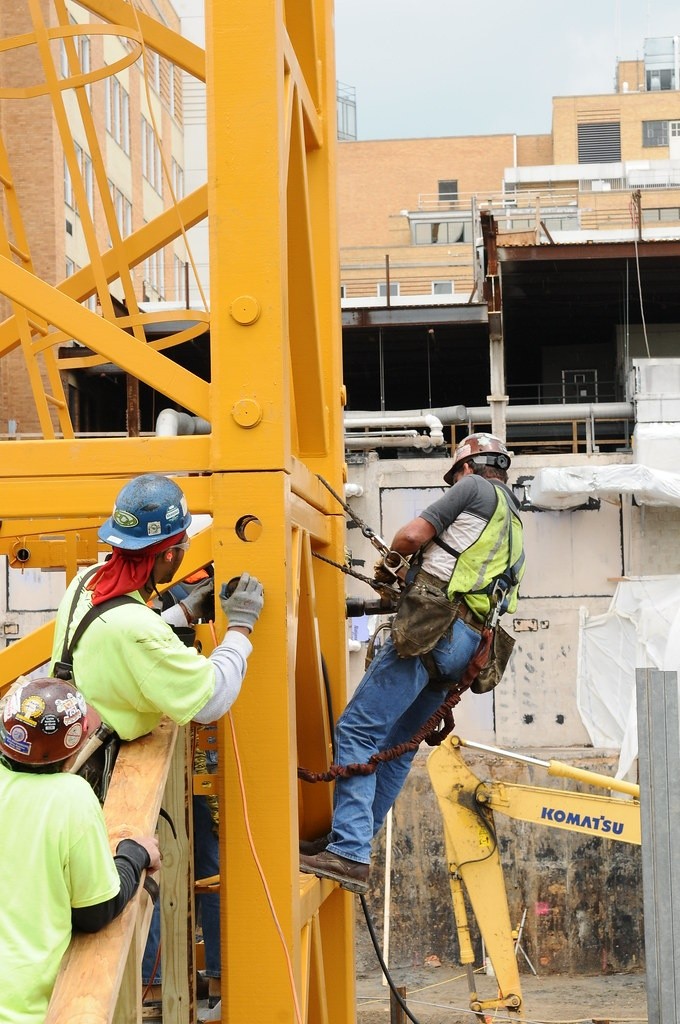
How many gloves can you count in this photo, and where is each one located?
[370,555,400,603]
[219,572,264,634]
[180,577,214,621]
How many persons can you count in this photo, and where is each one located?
[0,474,264,1024]
[299,433,528,893]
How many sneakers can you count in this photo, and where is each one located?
[141,983,163,1008]
[299,836,330,856]
[208,977,221,1009]
[299,850,369,895]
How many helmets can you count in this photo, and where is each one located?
[97,474,191,550]
[0,678,101,765]
[443,433,512,486]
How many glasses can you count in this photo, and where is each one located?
[167,537,191,552]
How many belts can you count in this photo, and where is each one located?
[457,603,485,632]
